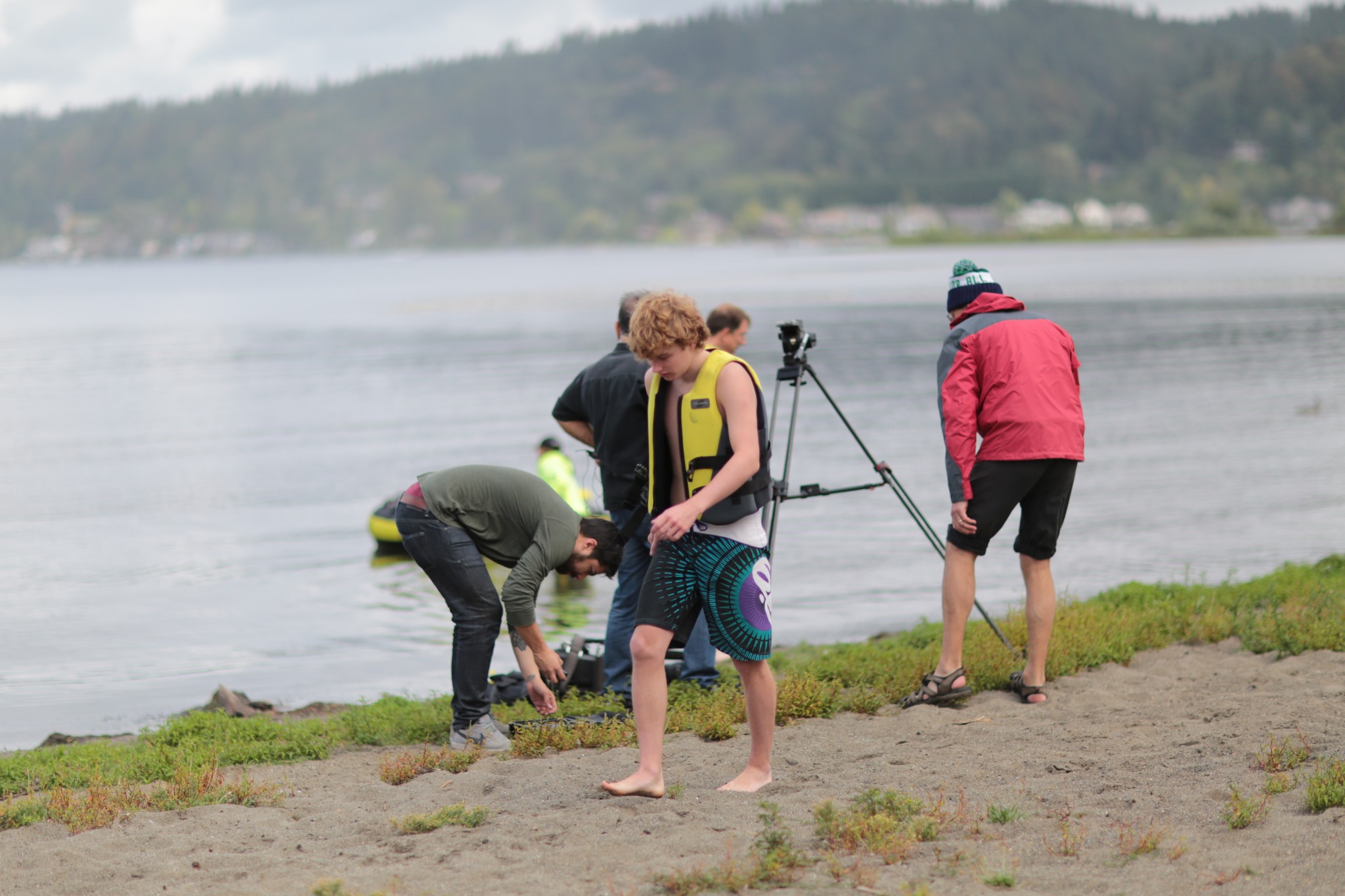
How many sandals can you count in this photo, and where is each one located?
[1009,671,1047,704]
[900,667,971,709]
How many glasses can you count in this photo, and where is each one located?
[947,311,953,321]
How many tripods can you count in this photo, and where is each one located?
[761,365,1008,647]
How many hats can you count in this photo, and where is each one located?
[947,259,1003,313]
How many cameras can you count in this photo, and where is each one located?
[775,323,816,358]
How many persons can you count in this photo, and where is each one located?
[524,292,778,799]
[392,464,624,751]
[898,261,1085,712]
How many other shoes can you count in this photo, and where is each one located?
[452,713,511,752]
[490,713,509,734]
